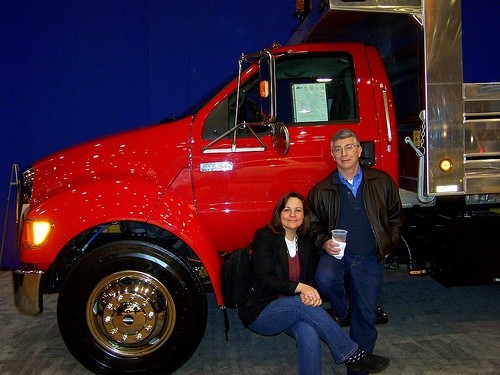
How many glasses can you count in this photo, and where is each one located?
[331,144,359,155]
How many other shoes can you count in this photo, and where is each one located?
[344,348,390,374]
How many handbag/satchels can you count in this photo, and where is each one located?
[216,241,265,342]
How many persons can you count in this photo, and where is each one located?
[238,192,390,375]
[305,130,403,375]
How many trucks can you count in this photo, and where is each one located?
[0,0,500,375]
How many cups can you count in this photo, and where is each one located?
[331,229,347,243]
